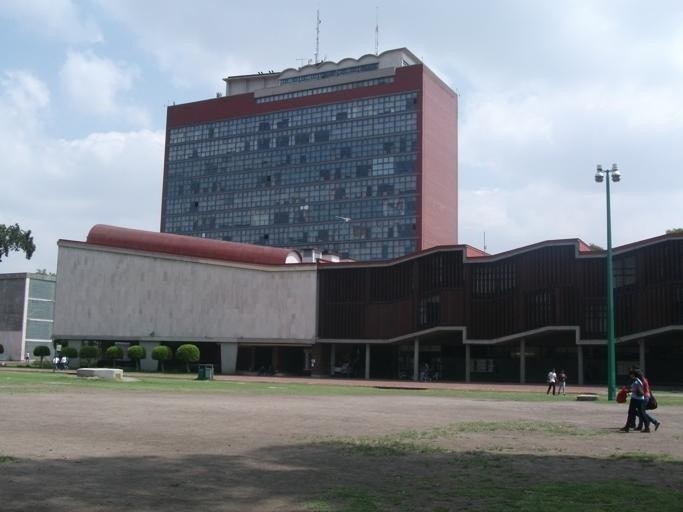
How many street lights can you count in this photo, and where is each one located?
[591,160,628,402]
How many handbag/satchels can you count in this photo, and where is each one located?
[635,383,645,397]
[646,395,658,410]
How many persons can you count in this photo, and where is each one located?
[59,355,67,370]
[25,351,30,362]
[546,367,557,396]
[557,367,568,395]
[53,356,59,373]
[617,367,660,433]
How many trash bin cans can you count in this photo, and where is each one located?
[198,364,215,380]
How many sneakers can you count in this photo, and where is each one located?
[655,422,660,430]
[620,423,651,433]
